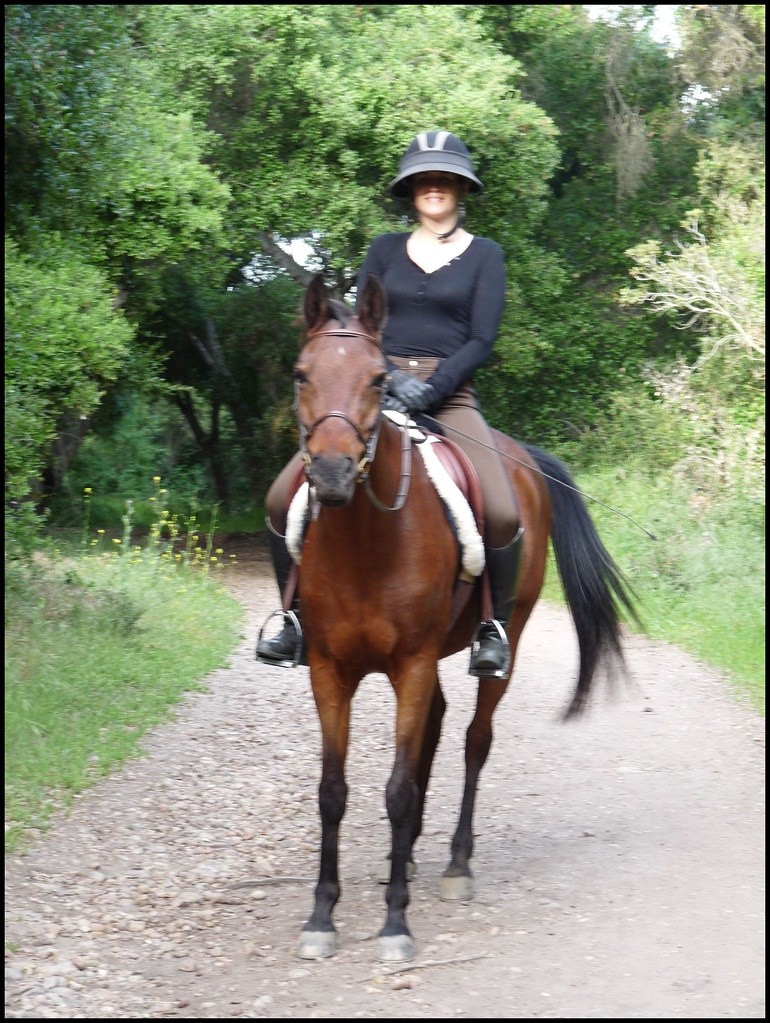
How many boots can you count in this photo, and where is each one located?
[470,527,524,679]
[256,515,310,666]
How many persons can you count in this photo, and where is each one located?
[254,130,521,673]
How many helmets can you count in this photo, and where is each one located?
[389,132,484,198]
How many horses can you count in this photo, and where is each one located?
[292,271,644,967]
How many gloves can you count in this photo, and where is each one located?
[384,369,438,411]
[382,384,437,414]
